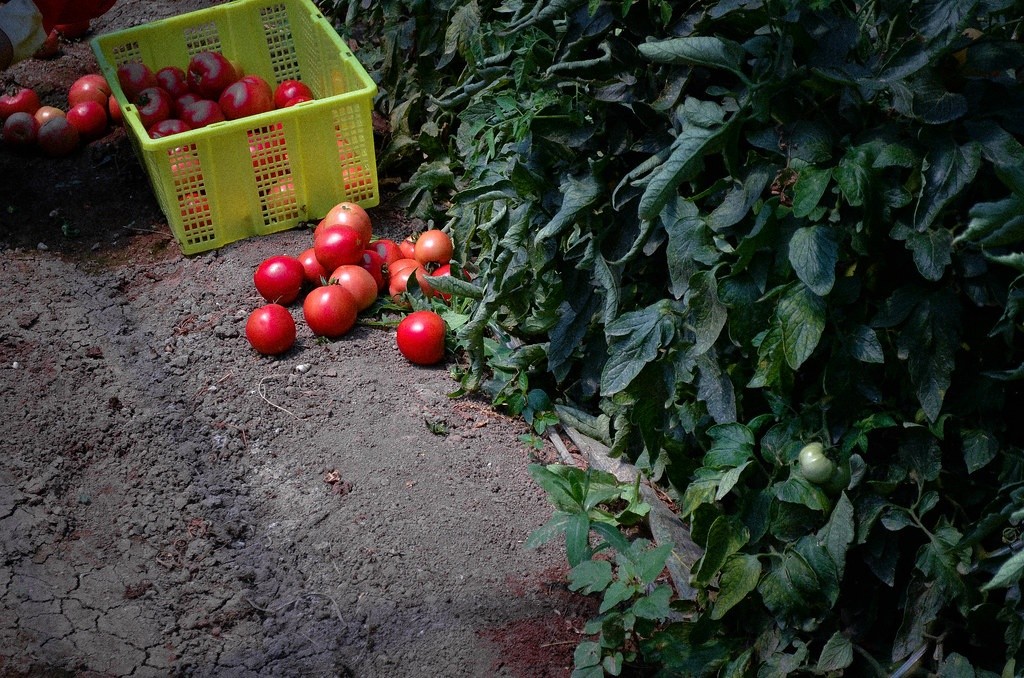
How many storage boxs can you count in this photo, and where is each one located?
[90,0,381,259]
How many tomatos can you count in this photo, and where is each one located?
[245,202,471,365]
[0,74,124,153]
[33,19,91,58]
[119,51,372,230]
